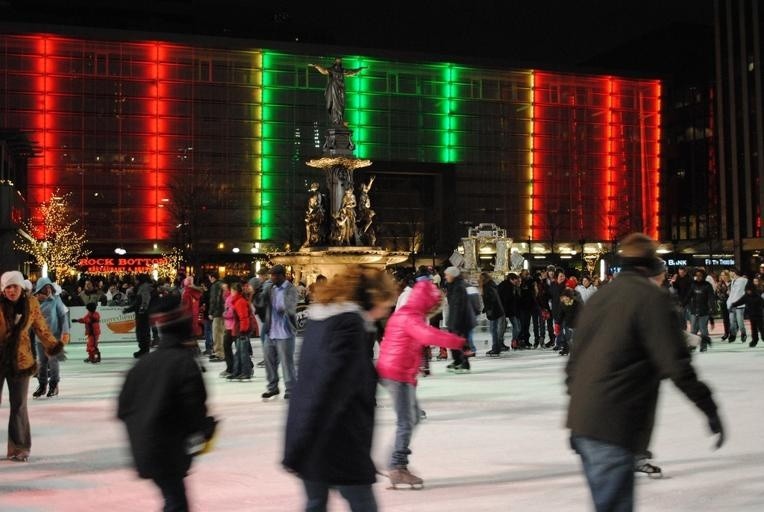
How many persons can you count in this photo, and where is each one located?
[72,272,122,306]
[301,179,325,246]
[262,263,298,402]
[219,276,236,377]
[559,287,584,357]
[402,264,442,279]
[151,323,160,349]
[227,284,258,379]
[257,268,272,368]
[726,265,748,344]
[499,272,517,352]
[72,302,101,364]
[295,280,306,305]
[575,276,597,303]
[207,271,224,362]
[417,306,448,377]
[279,272,398,512]
[565,233,725,512]
[116,297,219,512]
[181,276,203,337]
[122,273,150,359]
[201,282,213,354]
[671,267,692,304]
[308,56,368,127]
[478,272,504,359]
[518,264,565,356]
[715,271,731,340]
[151,276,181,297]
[731,281,764,348]
[0,271,68,462]
[329,174,378,248]
[745,263,764,295]
[32,277,70,399]
[683,267,711,352]
[377,279,469,491]
[443,266,477,373]
[247,278,259,301]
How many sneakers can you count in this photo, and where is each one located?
[7,447,33,466]
[46,382,60,395]
[386,465,425,483]
[30,382,46,397]
[453,360,471,372]
[219,364,252,380]
[633,461,662,474]
[446,359,461,368]
[485,348,499,355]
[261,387,281,398]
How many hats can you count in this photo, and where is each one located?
[693,264,706,276]
[24,277,34,291]
[265,264,286,275]
[0,270,24,293]
[645,257,670,279]
[182,275,194,286]
[216,274,233,285]
[255,265,268,274]
[443,266,460,279]
[563,276,578,290]
